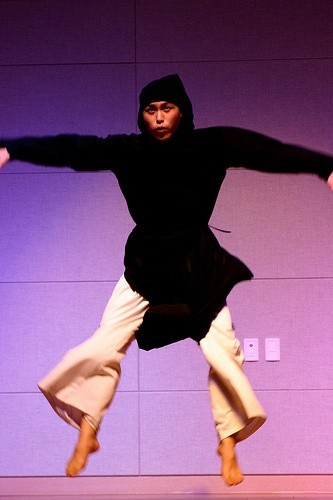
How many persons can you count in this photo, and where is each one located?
[0,71,333,490]
